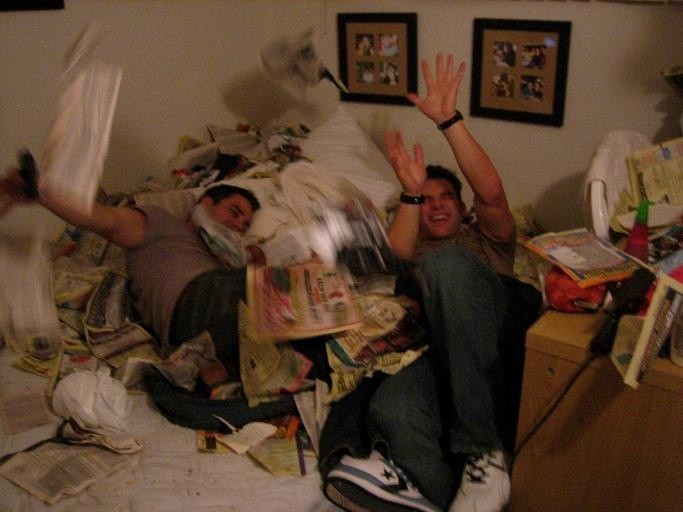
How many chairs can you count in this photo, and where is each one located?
[588,124,656,243]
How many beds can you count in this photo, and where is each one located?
[3,200,424,511]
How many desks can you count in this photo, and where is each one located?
[497,303,683,512]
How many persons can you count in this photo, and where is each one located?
[494,41,547,102]
[0,147,441,511]
[354,34,400,88]
[317,52,516,512]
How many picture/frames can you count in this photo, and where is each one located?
[335,10,418,107]
[468,14,574,130]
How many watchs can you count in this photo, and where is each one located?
[437,109,463,130]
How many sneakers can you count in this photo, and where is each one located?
[324,455,443,512]
[449,449,511,512]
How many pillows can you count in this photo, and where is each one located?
[296,101,404,214]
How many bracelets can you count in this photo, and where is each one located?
[398,193,425,204]
